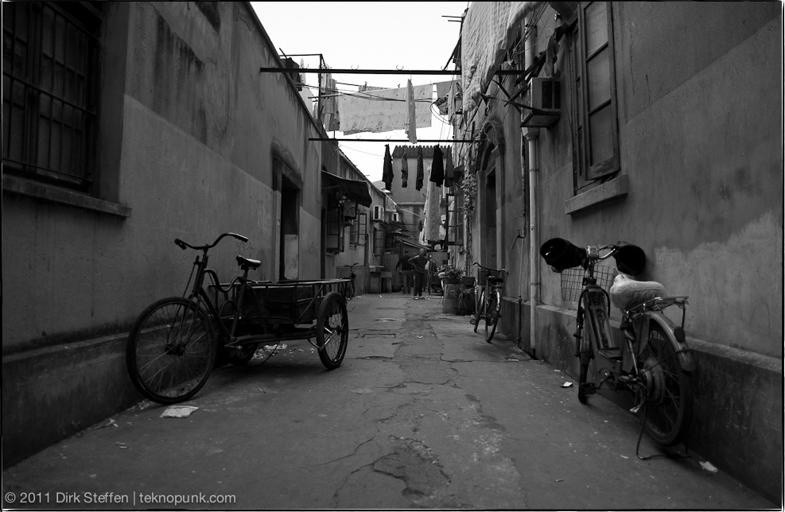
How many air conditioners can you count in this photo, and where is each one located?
[391,212,400,222]
[372,204,385,221]
[521,77,561,128]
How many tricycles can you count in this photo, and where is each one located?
[126,232,351,405]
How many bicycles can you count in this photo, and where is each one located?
[470,262,506,342]
[337,262,359,298]
[540,238,702,461]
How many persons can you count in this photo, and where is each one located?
[395,250,413,295]
[407,248,427,300]
